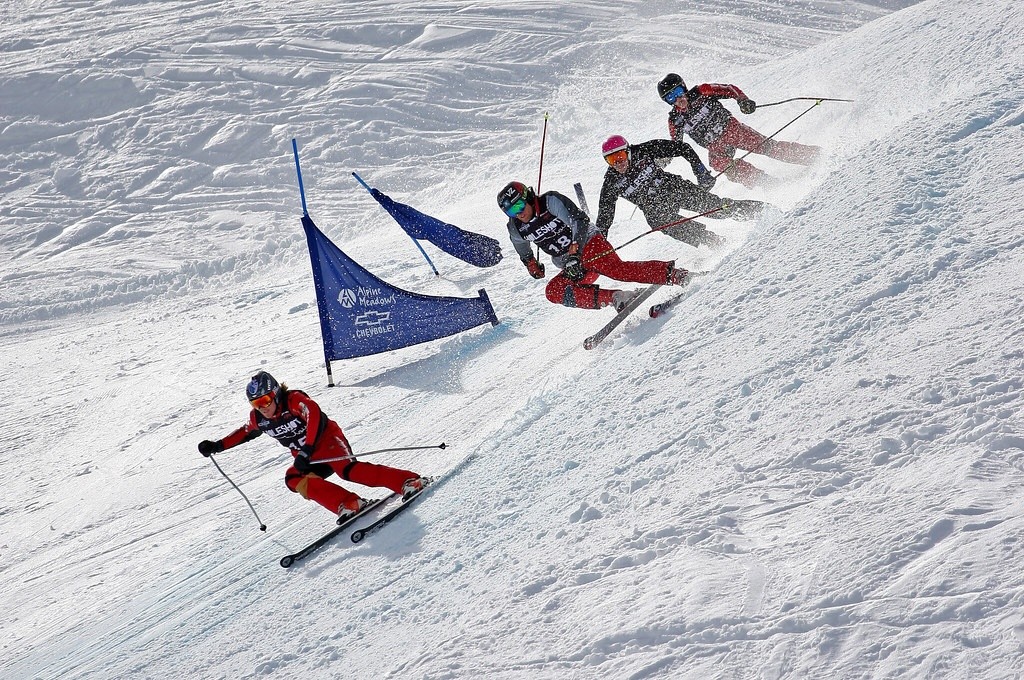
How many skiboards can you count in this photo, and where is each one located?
[279,456,473,569]
[582,281,712,349]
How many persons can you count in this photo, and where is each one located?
[596,134,772,249]
[497,181,689,314]
[198,369,428,525]
[658,72,823,190]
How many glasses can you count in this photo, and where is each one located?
[604,149,627,166]
[663,84,685,106]
[503,198,526,217]
[250,394,272,411]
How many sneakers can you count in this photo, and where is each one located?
[402,475,431,502]
[670,267,690,287]
[335,496,368,526]
[611,290,635,314]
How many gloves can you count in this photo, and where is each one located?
[527,257,545,279]
[736,98,756,114]
[696,167,716,192]
[563,253,584,282]
[294,444,316,471]
[198,440,223,458]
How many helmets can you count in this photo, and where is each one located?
[658,73,686,106]
[602,134,628,165]
[497,182,534,213]
[245,371,280,404]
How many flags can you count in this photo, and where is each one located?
[302,215,498,362]
[372,189,502,267]
